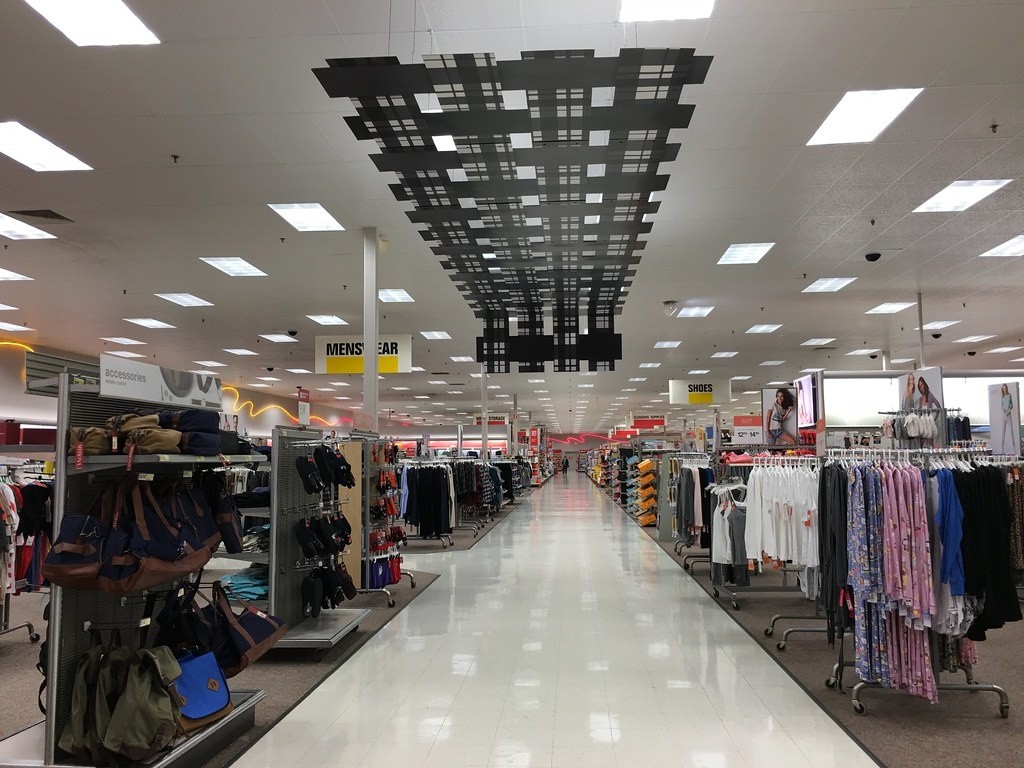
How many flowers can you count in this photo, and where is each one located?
[824,440,1024,473]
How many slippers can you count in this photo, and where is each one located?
[362,555,401,591]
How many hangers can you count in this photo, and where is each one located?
[884,408,965,421]
[749,457,820,479]
[398,458,518,469]
[680,458,711,470]
[709,476,748,494]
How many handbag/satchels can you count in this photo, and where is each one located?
[37,408,289,765]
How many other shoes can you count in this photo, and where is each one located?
[295,446,358,619]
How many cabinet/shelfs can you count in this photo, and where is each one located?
[0,442,266,768]
[597,463,611,488]
[611,458,627,508]
[518,446,555,487]
[512,468,525,497]
[626,451,661,527]
[211,428,381,662]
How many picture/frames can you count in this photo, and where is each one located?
[762,387,800,446]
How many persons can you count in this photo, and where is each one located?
[901,373,915,410]
[798,380,812,425]
[918,376,941,422]
[765,388,796,445]
[1000,383,1015,454]
[561,456,570,479]
